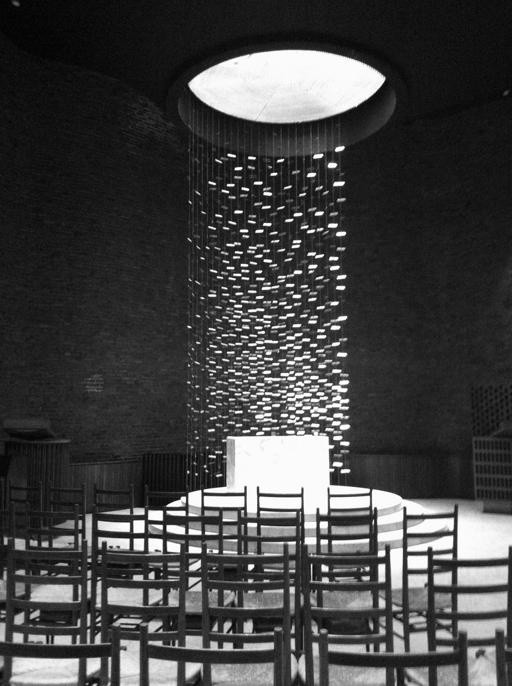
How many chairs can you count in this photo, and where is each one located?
[257,486,305,592]
[310,508,381,652]
[319,628,468,686]
[7,535,101,686]
[48,479,100,577]
[202,541,298,686]
[298,544,400,686]
[403,546,512,686]
[496,626,512,686]
[0,626,123,686]
[91,503,167,645]
[379,503,459,652]
[234,507,304,660]
[144,486,201,580]
[100,538,200,686]
[327,487,373,582]
[25,502,79,602]
[7,480,68,577]
[162,506,236,650]
[201,486,248,593]
[92,483,134,579]
[139,621,283,686]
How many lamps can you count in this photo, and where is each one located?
[175,43,399,497]
